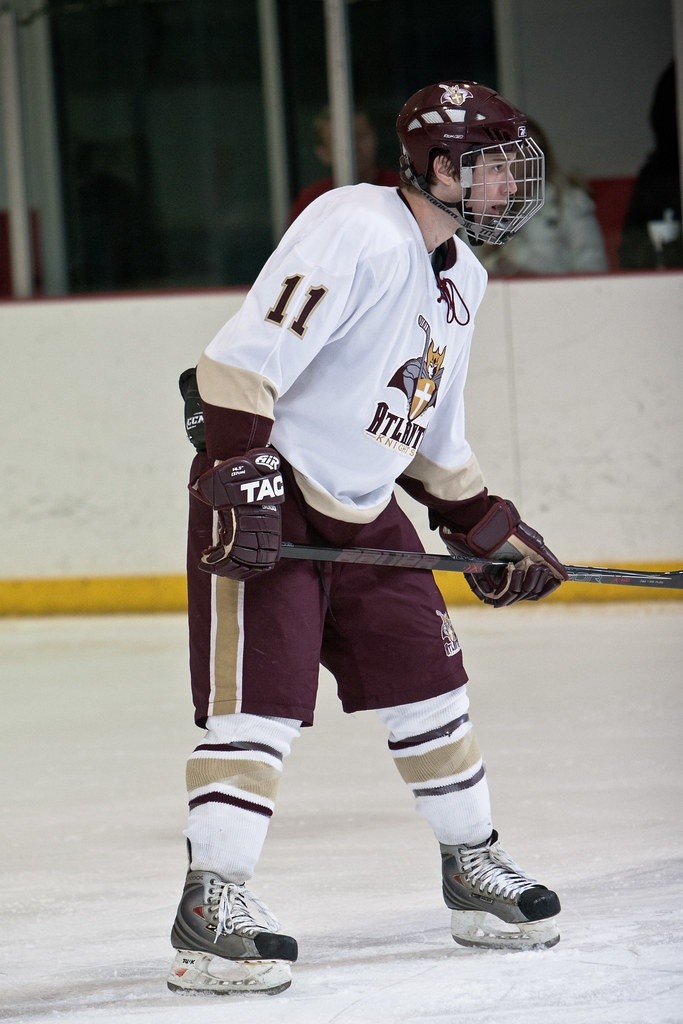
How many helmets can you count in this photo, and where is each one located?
[396,80,545,247]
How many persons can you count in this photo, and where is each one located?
[291,57,683,277]
[169,80,569,959]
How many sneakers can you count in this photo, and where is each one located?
[439,829,561,949]
[167,870,298,996]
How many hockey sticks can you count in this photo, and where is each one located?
[274,538,683,592]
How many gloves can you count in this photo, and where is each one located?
[187,448,286,582]
[439,496,569,608]
